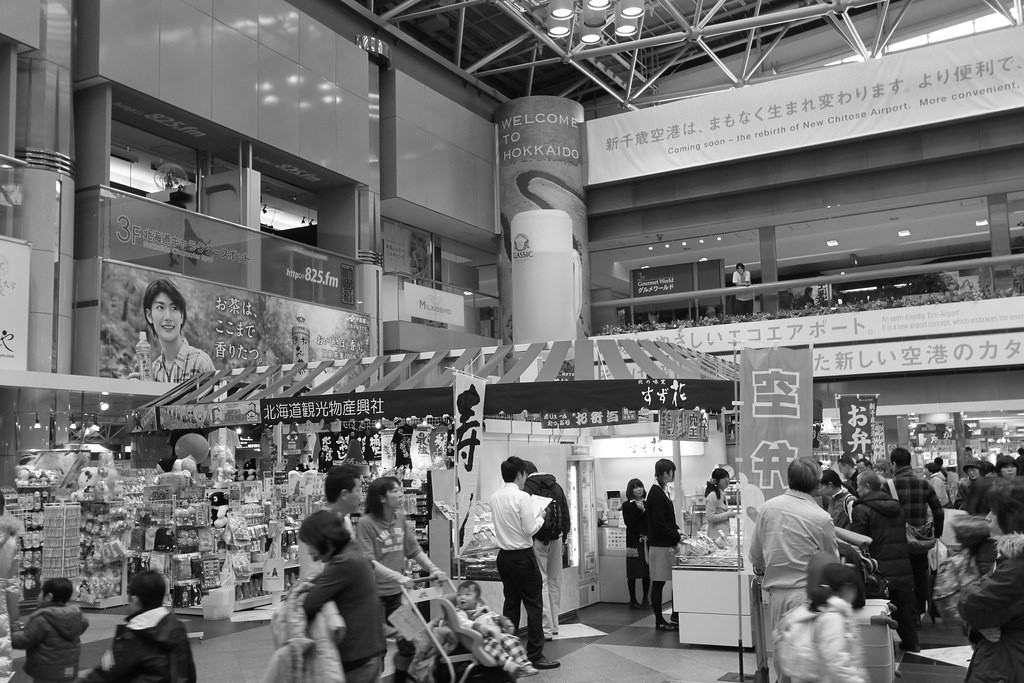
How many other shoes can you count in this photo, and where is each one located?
[629,602,643,609]
[655,616,679,631]
[532,655,560,668]
[642,600,649,605]
[519,666,538,676]
[899,643,920,653]
[670,612,679,622]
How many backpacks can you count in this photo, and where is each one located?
[526,479,562,545]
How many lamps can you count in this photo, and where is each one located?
[309,219,315,226]
[262,205,268,214]
[92,414,100,431]
[100,401,109,410]
[301,217,306,224]
[70,414,77,429]
[34,413,42,429]
[547,0,645,46]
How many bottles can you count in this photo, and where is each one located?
[292,313,310,382]
[135,331,153,382]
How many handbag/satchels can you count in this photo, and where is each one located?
[928,538,948,571]
[905,522,935,549]
[625,547,639,558]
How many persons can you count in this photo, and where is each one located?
[732,263,753,315]
[748,447,1024,683]
[83,570,196,683]
[453,580,539,680]
[796,287,814,309]
[522,461,570,640]
[646,459,686,631]
[129,275,215,385]
[0,491,91,683]
[300,510,388,683]
[621,478,651,610]
[489,456,560,670]
[706,469,736,541]
[300,463,364,581]
[355,476,447,683]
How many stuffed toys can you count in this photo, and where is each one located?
[70,467,99,501]
[14,465,58,488]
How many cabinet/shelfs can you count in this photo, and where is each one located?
[226,479,273,611]
[270,494,418,609]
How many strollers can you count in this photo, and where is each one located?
[398,574,520,683]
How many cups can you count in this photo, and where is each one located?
[510,211,582,361]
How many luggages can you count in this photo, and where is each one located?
[925,535,943,623]
[848,599,901,683]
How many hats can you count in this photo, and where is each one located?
[963,458,981,474]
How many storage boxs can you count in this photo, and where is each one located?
[201,587,236,621]
